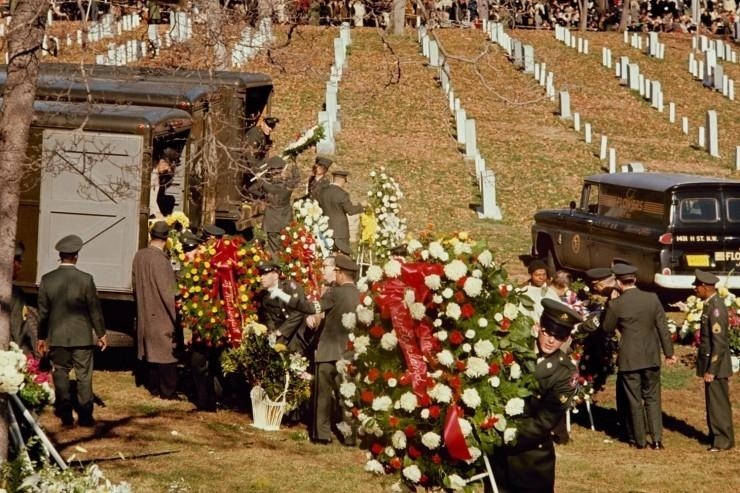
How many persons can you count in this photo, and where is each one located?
[320,0,740,45]
[483,258,677,493]
[691,269,735,451]
[131,117,367,446]
[37,234,107,413]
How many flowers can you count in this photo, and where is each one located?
[560,287,596,414]
[168,199,333,348]
[221,316,314,411]
[666,286,740,356]
[335,229,538,493]
[361,166,407,260]
[0,340,56,406]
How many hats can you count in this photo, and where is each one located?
[150,147,360,273]
[56,235,82,255]
[527,259,719,337]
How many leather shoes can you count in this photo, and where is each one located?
[637,439,721,451]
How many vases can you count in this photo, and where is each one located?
[249,386,284,430]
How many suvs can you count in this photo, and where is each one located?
[528,169,740,290]
